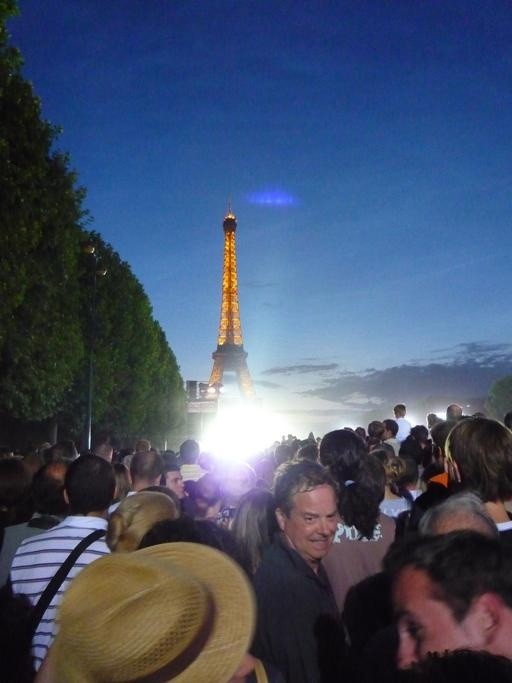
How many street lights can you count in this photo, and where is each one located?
[79,238,108,453]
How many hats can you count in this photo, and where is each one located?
[50,541,256,683]
